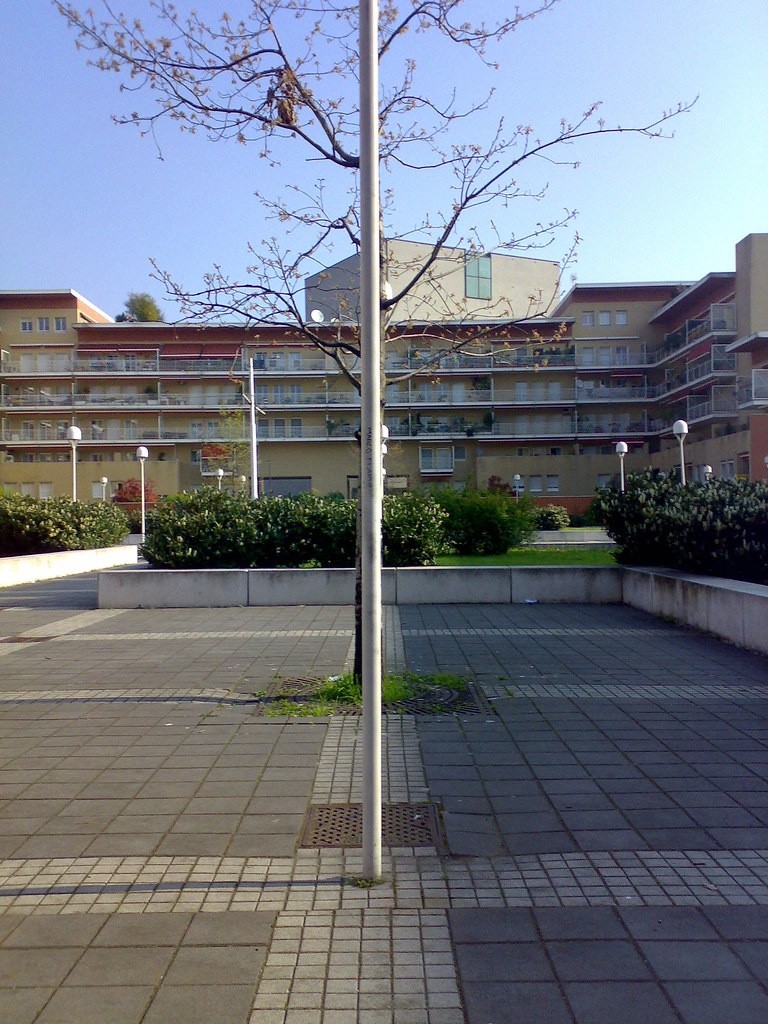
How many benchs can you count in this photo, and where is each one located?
[162,432,188,439]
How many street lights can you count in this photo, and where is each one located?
[704,465,712,485]
[673,420,688,485]
[100,477,107,501]
[136,447,149,544]
[216,468,223,490]
[239,475,246,489]
[67,426,81,502]
[616,442,628,495]
[514,474,520,503]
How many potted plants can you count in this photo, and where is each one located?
[145,387,158,405]
[74,389,86,406]
